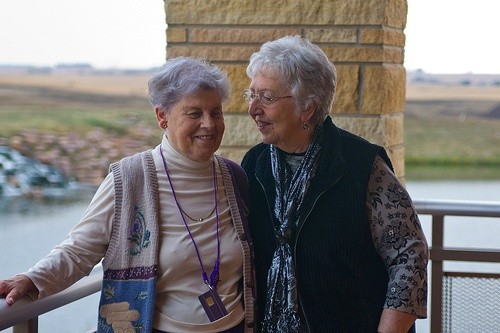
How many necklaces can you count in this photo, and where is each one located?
[168,152,218,222]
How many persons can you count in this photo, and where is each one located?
[240,35,428,333]
[1,56,254,333]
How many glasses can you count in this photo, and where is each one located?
[242,88,295,104]
